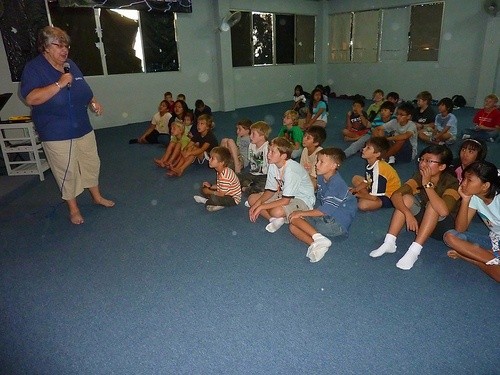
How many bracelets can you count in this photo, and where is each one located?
[209,184,212,189]
[56,82,61,89]
[360,115,363,116]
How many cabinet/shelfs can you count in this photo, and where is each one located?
[0,122,50,181]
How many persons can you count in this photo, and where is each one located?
[20,27,115,225]
[293,84,330,130]
[444,162,500,282]
[193,147,241,212]
[347,137,401,211]
[288,147,358,263]
[218,109,327,195]
[129,91,218,176]
[248,138,316,233]
[344,88,500,186]
[370,144,462,271]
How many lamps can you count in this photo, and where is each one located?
[216,9,241,33]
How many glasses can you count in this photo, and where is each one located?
[51,43,70,49]
[417,157,444,165]
[395,112,409,117]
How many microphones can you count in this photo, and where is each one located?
[63,62,71,89]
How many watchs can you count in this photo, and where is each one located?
[423,182,433,188]
